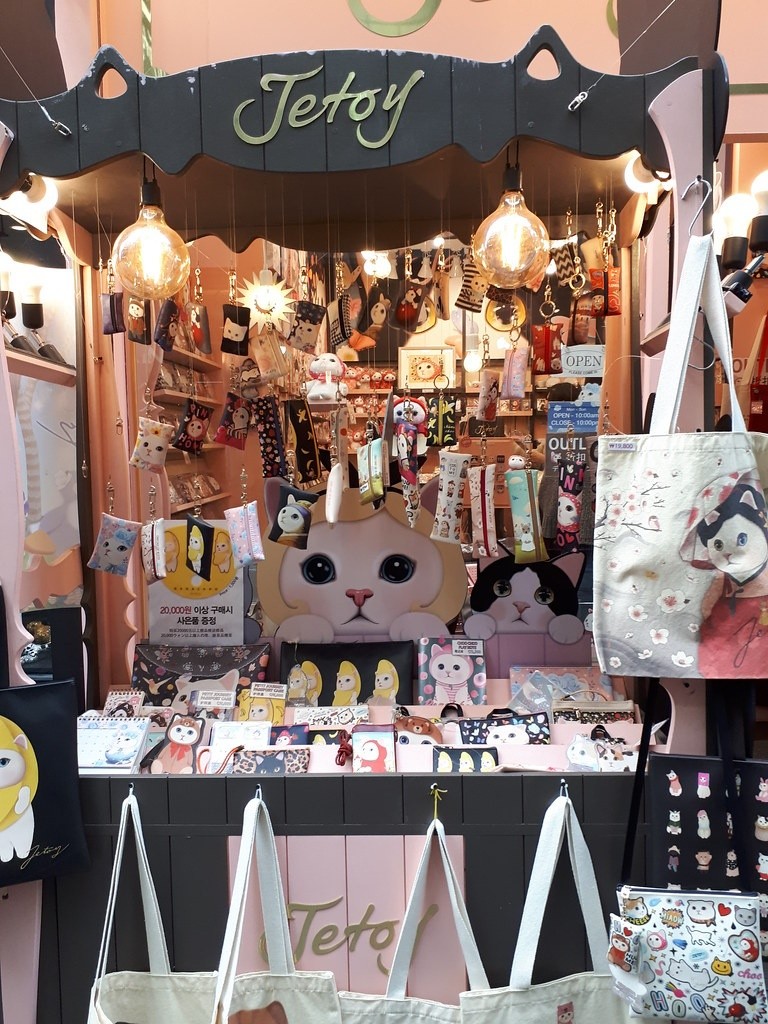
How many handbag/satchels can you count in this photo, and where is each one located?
[592,238,768,679]
[644,680,768,932]
[337,819,491,1024]
[77,637,644,777]
[87,795,219,1024]
[0,585,86,888]
[607,883,768,1024]
[210,797,342,1024]
[459,796,671,1024]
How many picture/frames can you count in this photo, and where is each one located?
[398,346,456,390]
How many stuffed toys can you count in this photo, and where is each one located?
[504,454,525,487]
[304,352,429,458]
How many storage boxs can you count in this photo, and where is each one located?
[460,438,535,507]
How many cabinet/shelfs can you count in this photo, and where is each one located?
[467,386,533,417]
[347,387,398,455]
[153,343,230,513]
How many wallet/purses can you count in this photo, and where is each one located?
[96,201,623,585]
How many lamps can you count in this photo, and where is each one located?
[472,141,550,290]
[709,170,768,280]
[113,154,193,299]
